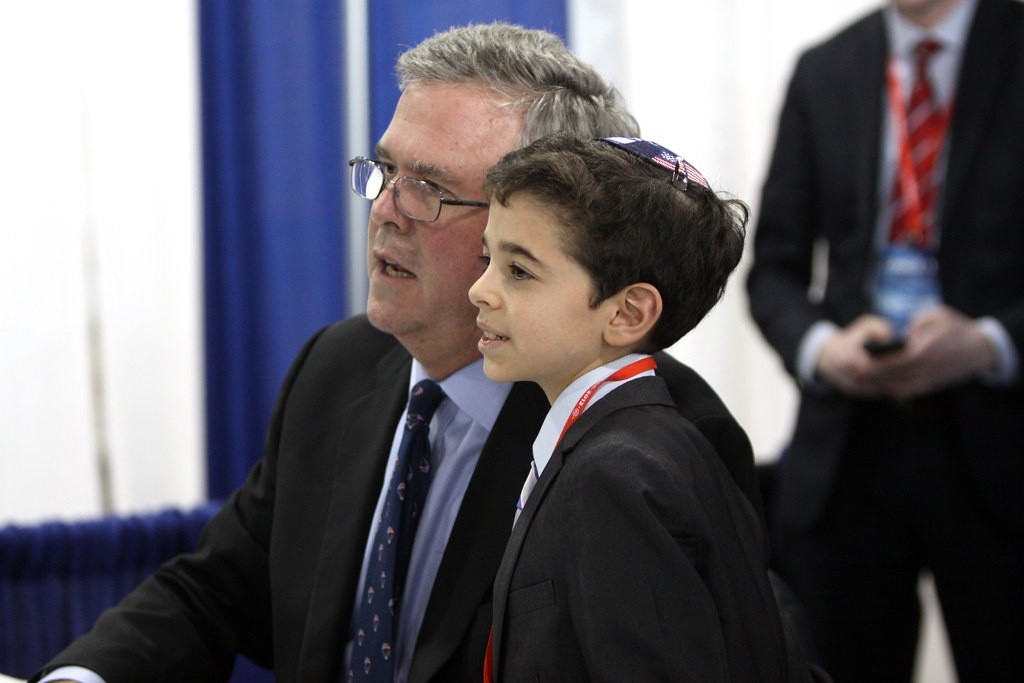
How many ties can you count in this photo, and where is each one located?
[349,378,447,683]
[889,41,942,250]
[511,462,538,531]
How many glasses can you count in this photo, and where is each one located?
[350,155,489,224]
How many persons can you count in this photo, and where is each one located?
[468,133,789,683]
[24,21,811,683]
[747,1,1024,681]
